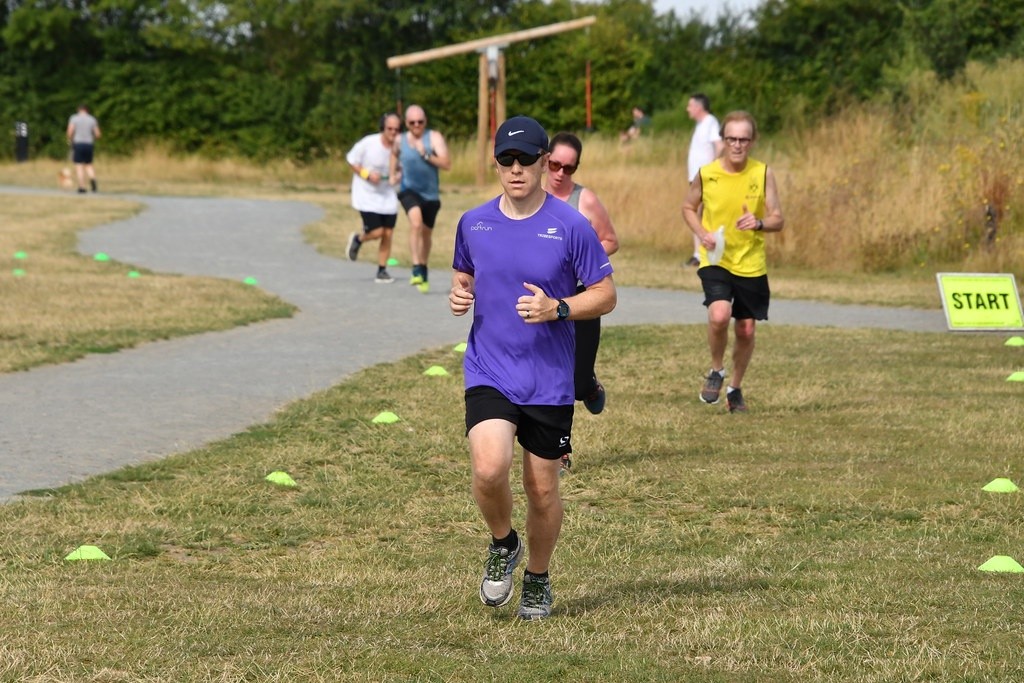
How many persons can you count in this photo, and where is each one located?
[68,105,101,192]
[632,108,651,138]
[686,94,725,265]
[540,132,619,415]
[682,112,784,412]
[389,105,450,291]
[449,116,616,620]
[346,112,403,283]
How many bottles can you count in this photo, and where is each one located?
[706,226,725,266]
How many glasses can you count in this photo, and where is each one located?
[406,119,426,127]
[723,136,752,148]
[386,126,400,132]
[495,150,543,166]
[548,159,579,175]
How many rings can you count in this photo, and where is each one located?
[526,310,529,318]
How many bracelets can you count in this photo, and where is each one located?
[758,220,763,231]
[422,152,430,160]
[359,169,369,179]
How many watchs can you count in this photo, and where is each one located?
[556,299,570,321]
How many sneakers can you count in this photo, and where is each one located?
[480,535,525,607]
[347,233,361,261]
[375,270,394,283]
[416,267,429,293]
[583,382,605,414]
[519,574,552,618]
[699,367,725,404]
[560,454,571,471]
[410,266,424,285]
[725,384,744,414]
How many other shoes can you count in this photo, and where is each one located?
[79,188,86,193]
[91,179,97,191]
[685,256,700,267]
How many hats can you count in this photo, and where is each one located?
[494,115,549,157]
